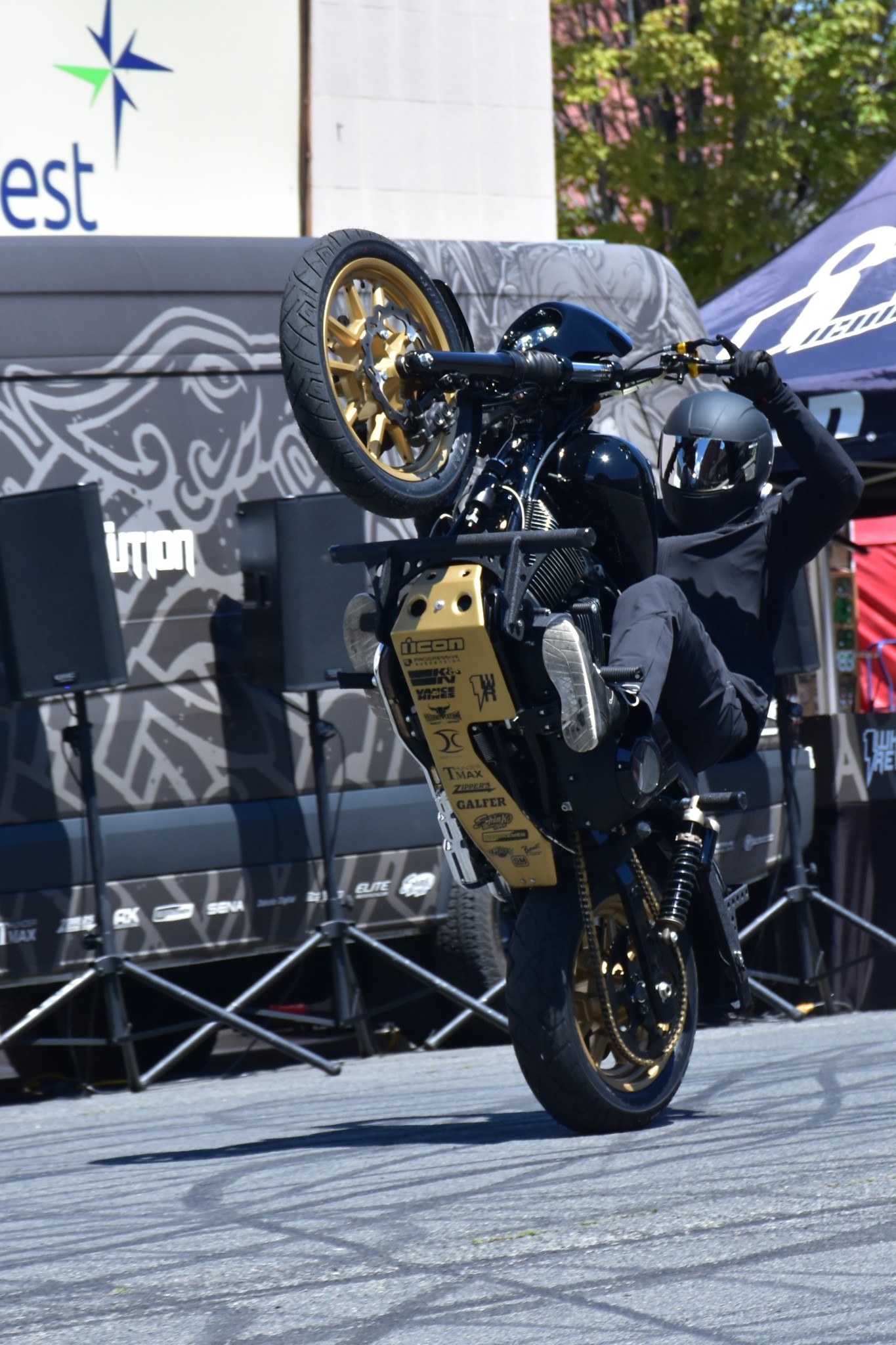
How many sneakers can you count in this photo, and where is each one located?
[342,593,392,726]
[543,616,622,752]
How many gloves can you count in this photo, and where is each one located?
[723,349,783,406]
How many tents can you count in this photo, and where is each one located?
[697,149,896,915]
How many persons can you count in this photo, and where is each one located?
[343,349,866,762]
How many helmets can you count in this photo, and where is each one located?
[658,389,774,527]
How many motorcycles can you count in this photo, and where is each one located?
[277,228,770,1136]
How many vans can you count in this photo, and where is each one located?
[0,231,817,1094]
